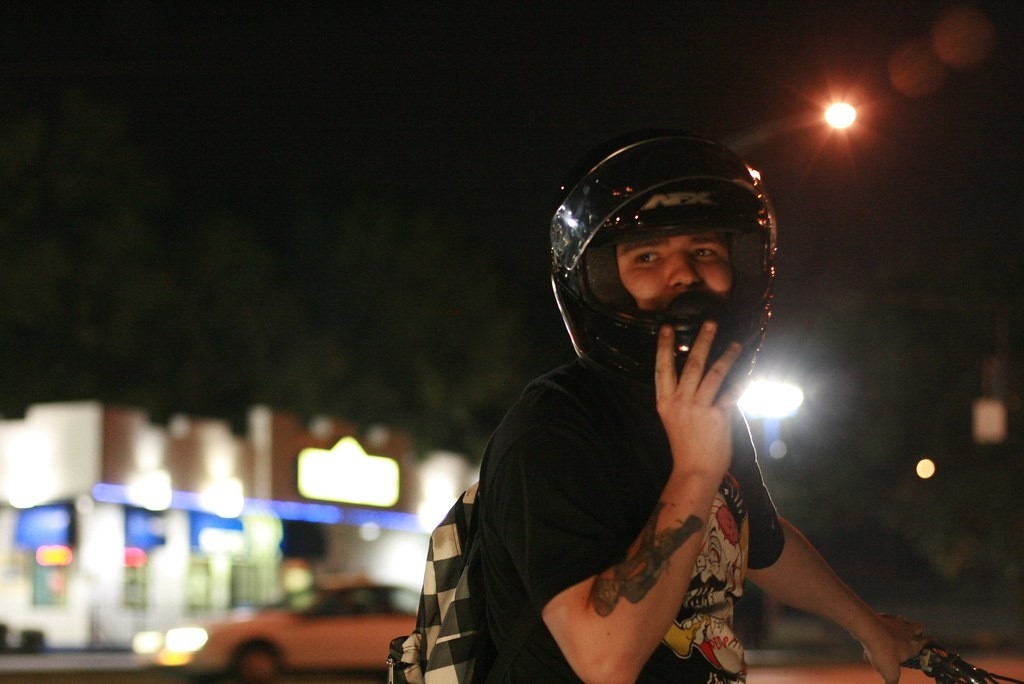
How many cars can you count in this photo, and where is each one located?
[151,574,420,684]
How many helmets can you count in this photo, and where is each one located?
[549,129,778,408]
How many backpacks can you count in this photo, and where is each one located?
[386,363,647,684]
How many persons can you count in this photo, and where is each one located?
[467,131,926,684]
